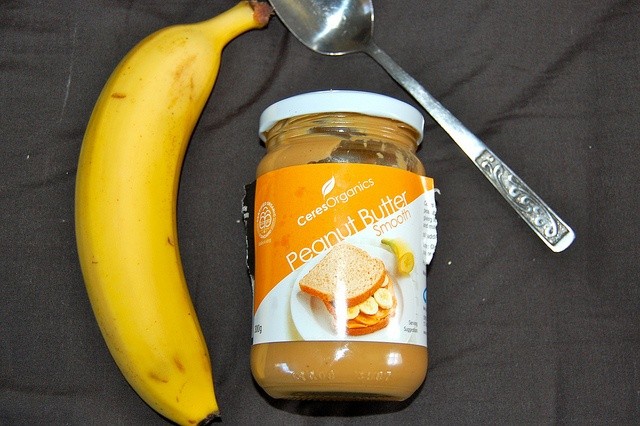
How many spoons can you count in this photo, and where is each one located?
[271,0,578,254]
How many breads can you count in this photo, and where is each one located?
[299,241,397,334]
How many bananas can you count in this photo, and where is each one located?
[380,236,414,274]
[73,0,274,426]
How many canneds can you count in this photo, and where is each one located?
[251,91,429,403]
